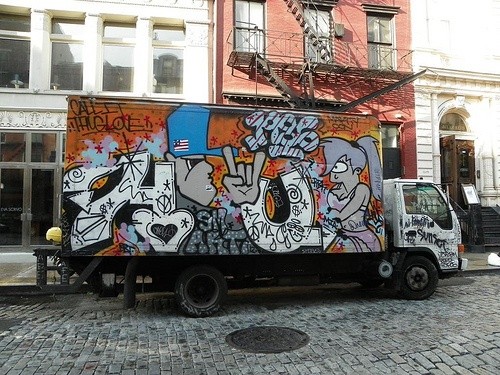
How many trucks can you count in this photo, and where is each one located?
[62,94,465,317]
